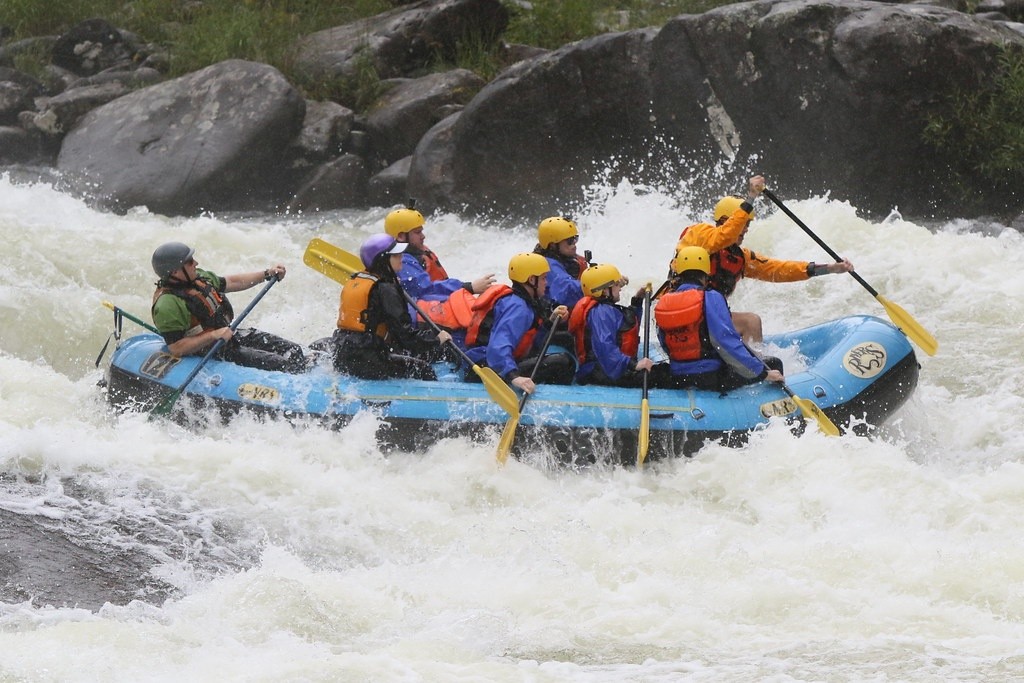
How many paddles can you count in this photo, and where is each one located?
[751,178,941,359]
[142,266,284,425]
[737,338,842,438]
[495,309,561,468]
[101,300,159,337]
[299,239,369,292]
[400,282,522,424]
[633,280,650,463]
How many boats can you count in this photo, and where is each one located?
[93,313,921,474]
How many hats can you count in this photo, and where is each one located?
[386,242,409,255]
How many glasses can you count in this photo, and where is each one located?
[563,236,578,244]
[184,257,194,266]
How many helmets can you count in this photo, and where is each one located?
[538,218,579,249]
[508,253,550,284]
[152,242,194,278]
[384,209,426,237]
[713,195,755,224]
[360,233,398,270]
[675,245,711,274]
[580,264,623,297]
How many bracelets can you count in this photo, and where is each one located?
[264,269,271,280]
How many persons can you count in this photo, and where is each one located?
[571,263,671,388]
[462,253,576,392]
[676,175,855,340]
[384,209,496,304]
[531,217,586,327]
[657,248,785,390]
[336,233,437,382]
[151,242,304,374]
